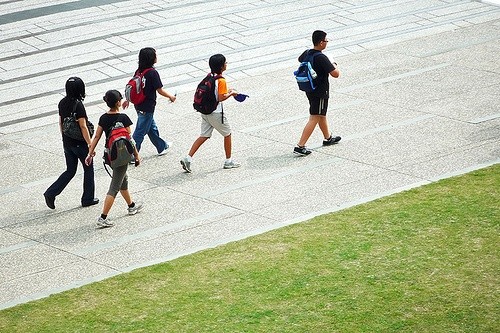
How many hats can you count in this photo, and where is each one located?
[234,94,250,102]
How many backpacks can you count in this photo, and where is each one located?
[103,114,136,178]
[294,49,324,92]
[125,67,155,105]
[192,72,226,115]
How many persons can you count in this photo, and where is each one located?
[85,90,143,227]
[127,47,176,163]
[293,30,342,155]
[44,77,99,209]
[180,54,241,172]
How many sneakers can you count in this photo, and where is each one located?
[323,135,341,145]
[180,156,192,173]
[224,159,241,169]
[127,201,145,215]
[97,216,115,227]
[293,143,312,156]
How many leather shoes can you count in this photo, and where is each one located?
[82,198,99,207]
[44,194,56,209]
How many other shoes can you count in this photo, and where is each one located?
[129,159,136,164]
[158,144,171,155]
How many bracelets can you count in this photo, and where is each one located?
[87,154,92,157]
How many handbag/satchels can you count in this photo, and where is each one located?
[63,100,94,141]
